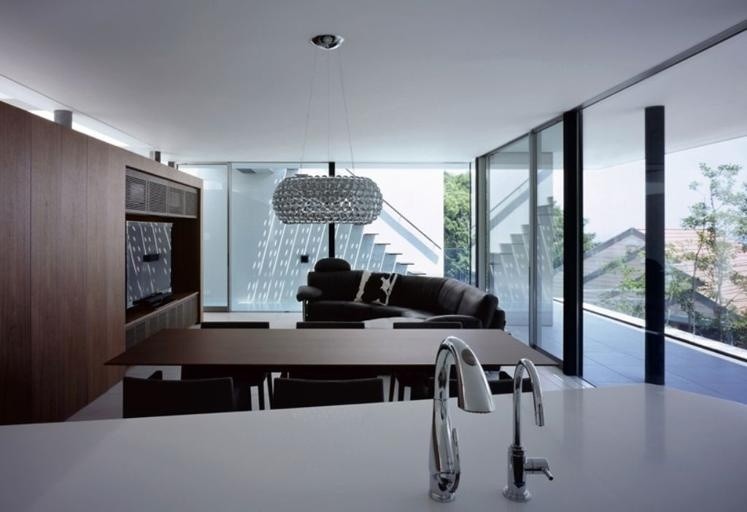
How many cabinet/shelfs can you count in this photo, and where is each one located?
[124,291,200,344]
[0,101,129,420]
[125,166,204,218]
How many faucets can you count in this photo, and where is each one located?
[503,357,545,501]
[426,334,496,503]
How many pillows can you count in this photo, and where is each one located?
[352,269,396,306]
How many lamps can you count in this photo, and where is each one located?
[271,32,381,227]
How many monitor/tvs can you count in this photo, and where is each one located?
[125,220,173,310]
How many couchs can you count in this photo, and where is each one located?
[296,257,507,369]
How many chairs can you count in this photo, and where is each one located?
[120,319,534,416]
